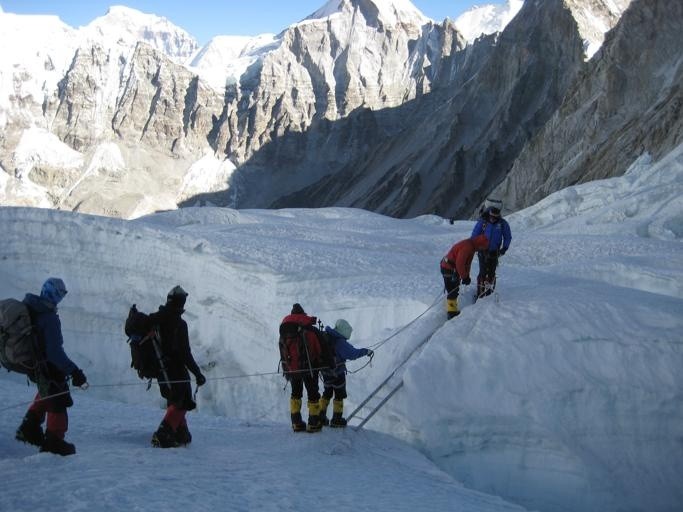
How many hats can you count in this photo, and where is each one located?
[335,319,353,340]
[167,284,188,308]
[40,278,68,305]
[291,304,304,314]
[490,207,500,217]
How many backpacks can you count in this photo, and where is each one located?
[0,298,40,374]
[280,321,309,378]
[125,304,162,378]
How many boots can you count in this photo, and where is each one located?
[17,410,45,446]
[39,430,76,456]
[292,410,347,433]
[151,420,191,448]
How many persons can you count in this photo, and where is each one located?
[281,304,337,434]
[320,319,373,427]
[470,207,512,300]
[439,235,491,320]
[149,285,206,448]
[15,277,86,454]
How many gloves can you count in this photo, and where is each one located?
[196,374,205,384]
[462,277,471,285]
[367,349,374,357]
[500,248,505,255]
[72,367,86,386]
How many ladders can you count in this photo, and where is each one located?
[345,334,434,433]
